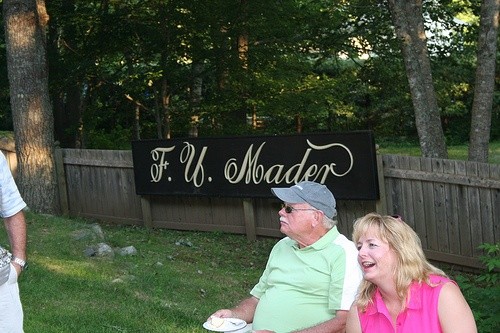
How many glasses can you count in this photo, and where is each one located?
[281,203,316,214]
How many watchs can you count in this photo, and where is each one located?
[11,256,27,271]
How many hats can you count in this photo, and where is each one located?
[270,181,336,219]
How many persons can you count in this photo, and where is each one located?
[205,182,364,333]
[0,149,27,333]
[346,213,478,333]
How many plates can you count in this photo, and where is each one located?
[203,318,247,332]
